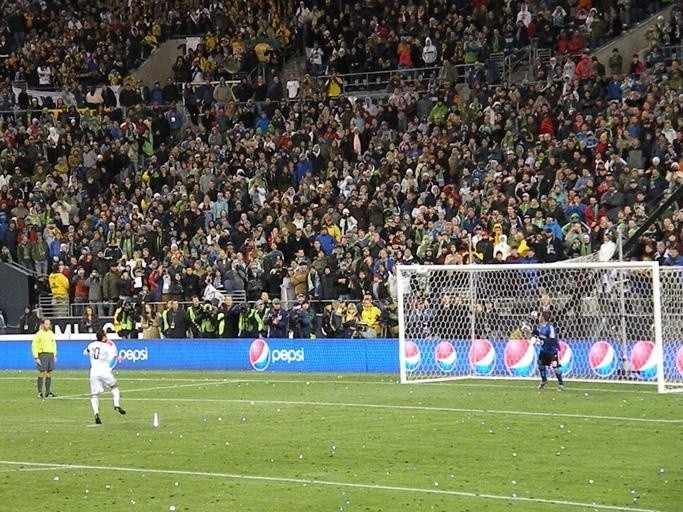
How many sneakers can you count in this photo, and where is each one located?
[557,384,565,392]
[95,414,100,425]
[537,382,549,391]
[113,406,127,415]
[37,391,56,398]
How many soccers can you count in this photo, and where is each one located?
[531,311,538,320]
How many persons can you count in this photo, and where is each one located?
[529,311,564,391]
[0,0,683,342]
[31,318,58,399]
[83,330,126,424]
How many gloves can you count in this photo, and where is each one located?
[521,325,532,334]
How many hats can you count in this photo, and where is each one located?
[6,98,683,304]
